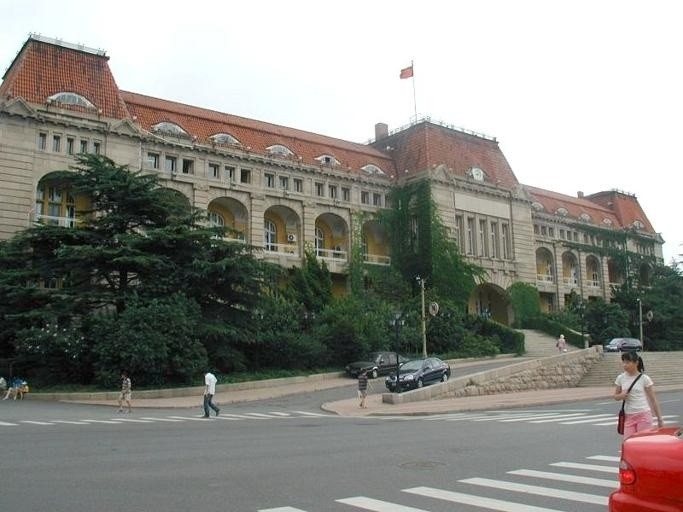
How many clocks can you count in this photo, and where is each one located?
[472,169,483,181]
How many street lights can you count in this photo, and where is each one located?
[574,300,587,349]
[388,310,408,392]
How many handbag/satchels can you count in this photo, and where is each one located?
[617,410,624,434]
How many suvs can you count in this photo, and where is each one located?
[603,337,643,353]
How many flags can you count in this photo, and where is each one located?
[400,66,413,79]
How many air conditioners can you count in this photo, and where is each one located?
[287,234,296,241]
[333,245,343,254]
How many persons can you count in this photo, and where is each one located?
[614,351,663,440]
[358,368,371,407]
[119,372,132,413]
[201,367,219,419]
[0,376,29,401]
[558,335,565,353]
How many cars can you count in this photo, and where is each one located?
[345,350,411,380]
[385,356,450,393]
